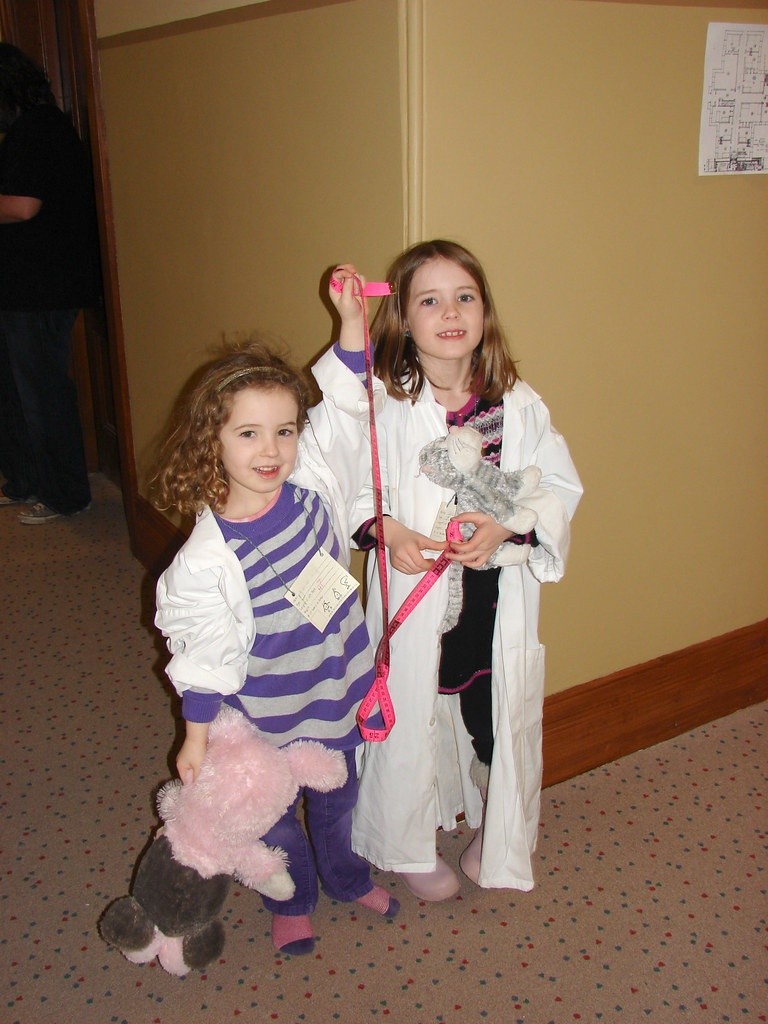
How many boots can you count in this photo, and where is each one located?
[459,755,492,884]
[393,851,459,902]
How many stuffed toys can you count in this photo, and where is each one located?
[418,425,544,568]
[99,704,347,979]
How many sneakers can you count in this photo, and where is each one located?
[0,488,41,504]
[18,499,91,525]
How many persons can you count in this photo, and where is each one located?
[147,263,400,956]
[341,239,584,901]
[1,44,95,523]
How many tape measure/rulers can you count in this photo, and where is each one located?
[329,265,469,743]
